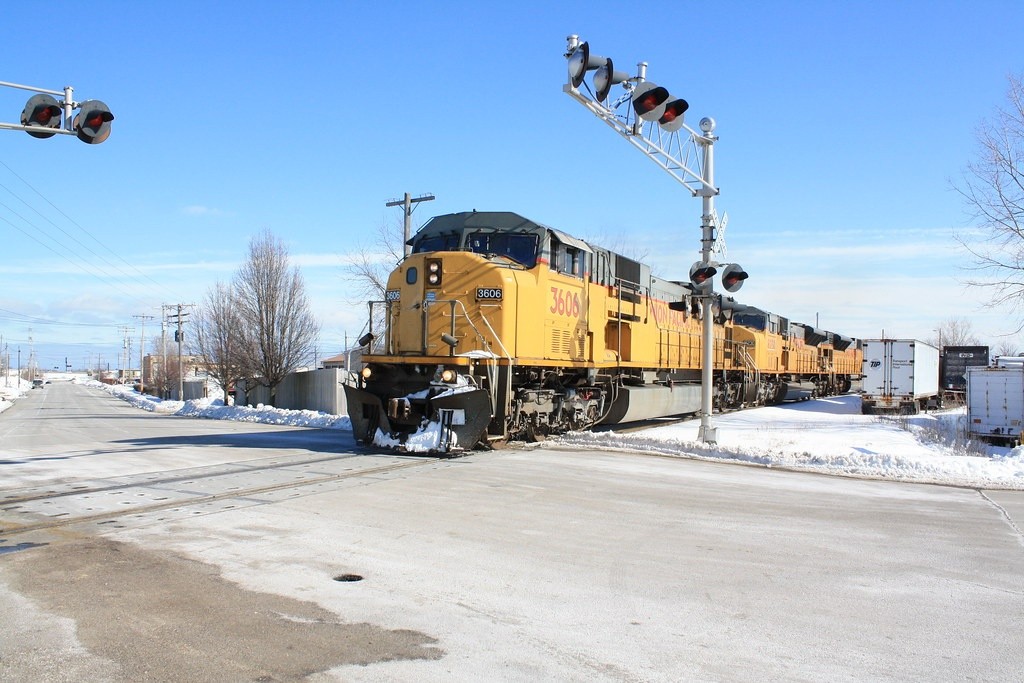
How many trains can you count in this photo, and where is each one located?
[339,208,863,460]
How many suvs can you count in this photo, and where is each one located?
[32,380,43,389]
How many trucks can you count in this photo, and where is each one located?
[943,345,989,400]
[991,356,1024,365]
[862,338,940,415]
[965,366,1024,448]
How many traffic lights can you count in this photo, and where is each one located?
[593,58,629,102]
[80,100,114,138]
[632,82,669,122]
[658,94,688,132]
[25,94,62,128]
[690,262,717,291]
[569,41,607,89]
[721,263,749,293]
